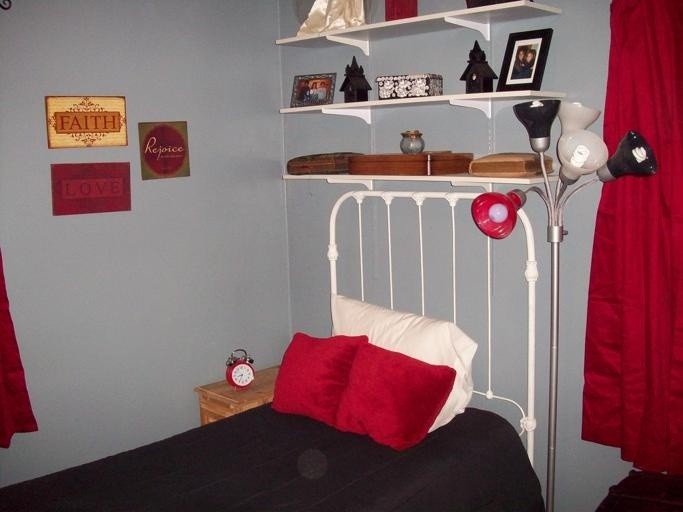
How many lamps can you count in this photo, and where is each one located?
[471,100,661,512]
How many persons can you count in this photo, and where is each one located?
[309,81,319,102]
[316,81,328,100]
[512,47,523,70]
[513,49,535,77]
[299,79,309,101]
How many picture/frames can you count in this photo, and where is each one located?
[495,28,553,92]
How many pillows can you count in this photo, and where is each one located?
[330,294,479,434]
[272,332,369,430]
[335,340,457,451]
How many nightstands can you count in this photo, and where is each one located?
[194,362,282,429]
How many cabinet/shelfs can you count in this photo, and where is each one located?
[276,1,566,191]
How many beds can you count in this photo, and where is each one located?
[0,188,567,512]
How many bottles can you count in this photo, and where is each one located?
[400,130,424,154]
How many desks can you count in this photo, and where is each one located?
[594,470,683,512]
